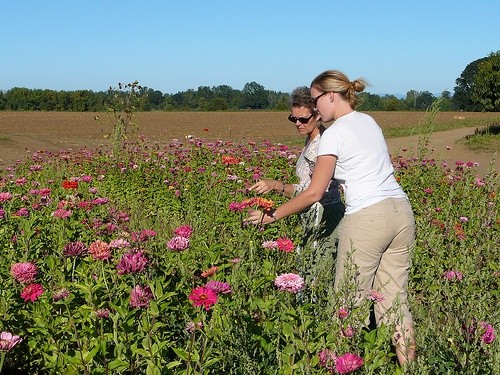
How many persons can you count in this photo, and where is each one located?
[244,71,415,366]
[249,87,345,249]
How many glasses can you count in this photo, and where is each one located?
[288,113,315,124]
[311,91,327,108]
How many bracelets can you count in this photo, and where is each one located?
[270,211,277,219]
[281,183,285,194]
